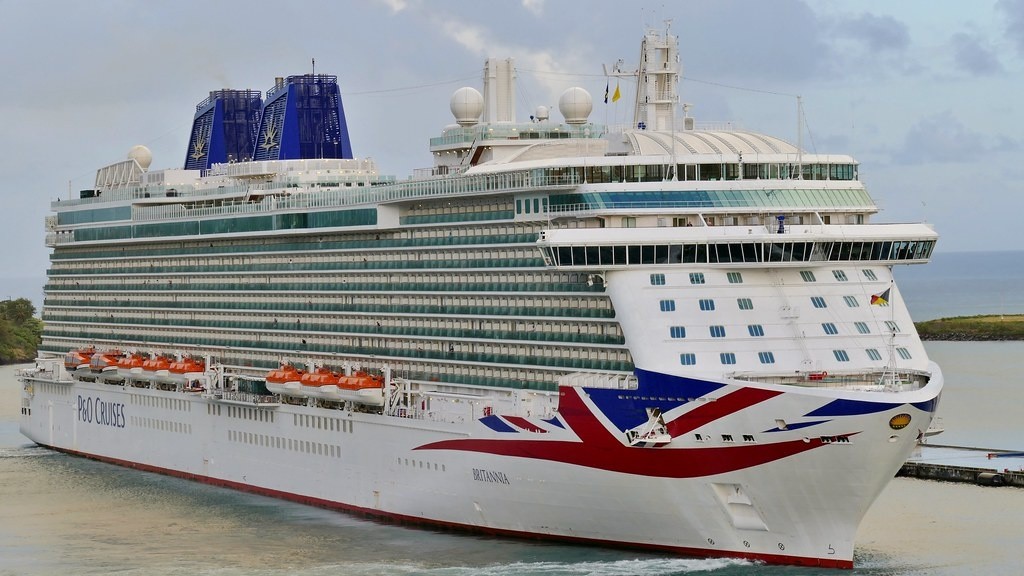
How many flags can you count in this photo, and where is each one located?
[871,288,890,304]
[604,83,608,104]
[612,87,620,102]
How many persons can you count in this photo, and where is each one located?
[376,235,379,240]
[274,318,277,322]
[363,258,366,261]
[450,346,454,351]
[377,322,380,326]
[297,319,300,323]
[93,344,95,348]
[302,339,306,344]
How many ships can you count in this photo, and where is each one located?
[15,12,945,571]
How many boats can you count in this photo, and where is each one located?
[925,417,944,436]
[65,349,92,380]
[118,356,145,381]
[170,361,205,385]
[301,367,342,402]
[266,364,302,399]
[90,353,121,380]
[144,358,174,383]
[337,371,385,407]
